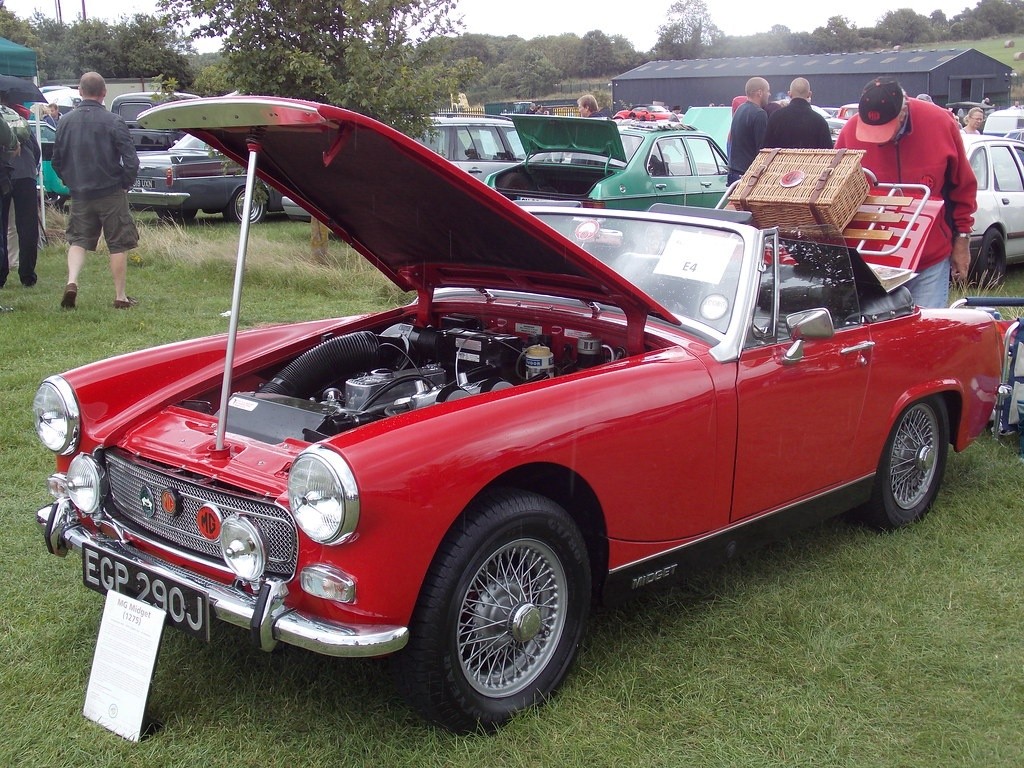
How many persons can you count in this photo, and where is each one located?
[959,107,984,135]
[725,77,771,185]
[628,111,640,121]
[832,76,978,307]
[534,104,546,115]
[576,94,613,120]
[649,114,655,121]
[917,93,963,128]
[0,104,63,288]
[761,78,832,149]
[984,96,992,107]
[670,105,682,123]
[639,114,647,121]
[763,102,781,118]
[726,96,749,160]
[1010,100,1021,109]
[526,103,536,114]
[49,71,140,310]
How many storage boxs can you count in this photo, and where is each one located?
[727,146,870,235]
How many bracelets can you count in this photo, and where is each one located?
[956,232,972,239]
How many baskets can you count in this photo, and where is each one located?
[728,148,870,235]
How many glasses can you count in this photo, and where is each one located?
[969,116,983,122]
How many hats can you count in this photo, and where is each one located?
[916,94,933,103]
[985,98,990,103]
[855,76,904,144]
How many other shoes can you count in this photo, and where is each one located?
[61,283,77,309]
[113,296,140,308]
[0,277,6,288]
[23,278,34,288]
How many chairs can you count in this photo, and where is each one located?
[742,265,858,349]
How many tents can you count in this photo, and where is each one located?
[0,37,48,232]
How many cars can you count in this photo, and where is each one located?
[482,107,733,261]
[959,133,1024,280]
[111,92,203,150]
[985,106,1024,141]
[30,97,1002,731]
[281,112,524,232]
[27,122,60,143]
[126,132,287,226]
[615,105,683,120]
